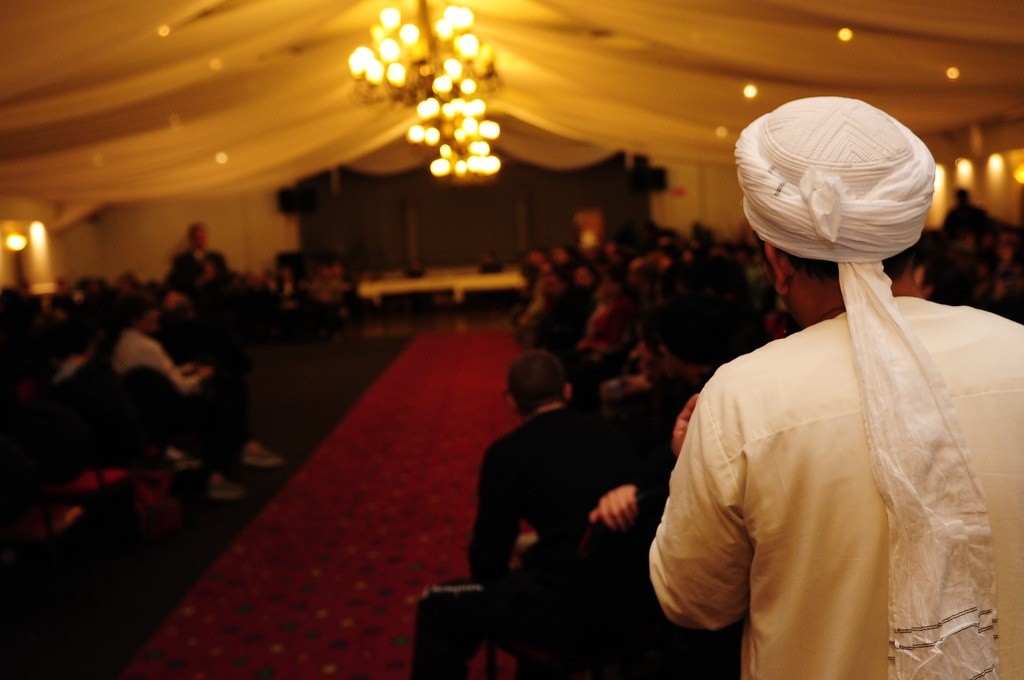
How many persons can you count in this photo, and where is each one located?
[649,95,1024,680]
[477,247,522,314]
[0,220,360,680]
[409,186,1024,680]
[408,258,426,316]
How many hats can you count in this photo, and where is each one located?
[735,97,937,264]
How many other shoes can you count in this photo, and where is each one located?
[241,445,286,468]
[208,479,246,499]
